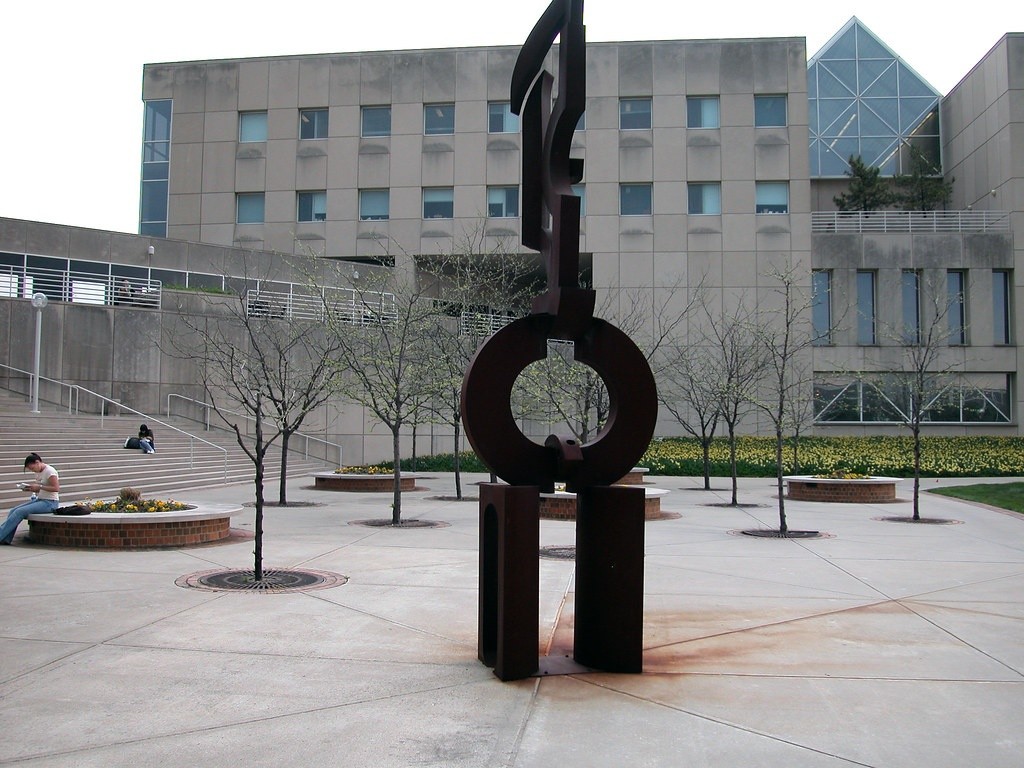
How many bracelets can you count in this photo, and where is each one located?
[40,484,42,488]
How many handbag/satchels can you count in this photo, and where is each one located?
[54,505,91,515]
[124,437,141,449]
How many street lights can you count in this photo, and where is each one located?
[31,293,47,413]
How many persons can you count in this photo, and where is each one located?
[138,424,156,454]
[0,452,60,545]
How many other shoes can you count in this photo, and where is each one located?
[150,450,154,454]
[0,539,10,545]
[147,450,150,454]
